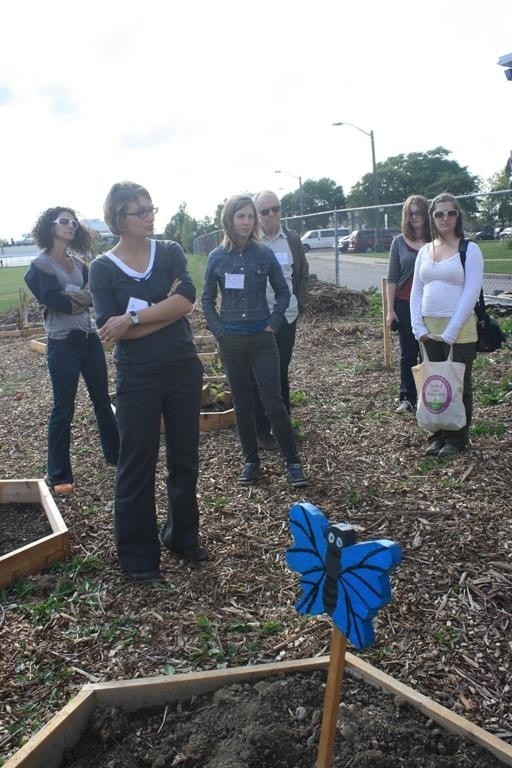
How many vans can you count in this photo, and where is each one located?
[349,229,401,253]
[298,227,351,252]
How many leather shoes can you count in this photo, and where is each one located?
[437,445,463,458]
[424,441,441,456]
[55,484,74,494]
[175,547,209,561]
[255,434,281,452]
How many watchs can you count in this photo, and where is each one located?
[127,310,140,328]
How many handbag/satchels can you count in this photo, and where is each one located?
[475,314,507,353]
[411,334,467,432]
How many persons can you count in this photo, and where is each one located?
[200,193,312,490]
[246,189,309,449]
[87,179,207,586]
[385,192,437,411]
[24,205,121,495]
[409,191,486,458]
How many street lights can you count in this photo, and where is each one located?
[330,120,386,253]
[275,169,306,231]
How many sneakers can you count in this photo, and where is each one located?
[287,463,308,486]
[394,401,414,413]
[237,463,265,485]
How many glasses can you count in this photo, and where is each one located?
[53,216,79,230]
[431,208,459,219]
[257,206,281,217]
[127,207,159,219]
[408,210,423,217]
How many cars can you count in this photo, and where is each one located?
[332,234,350,254]
[469,224,512,241]
